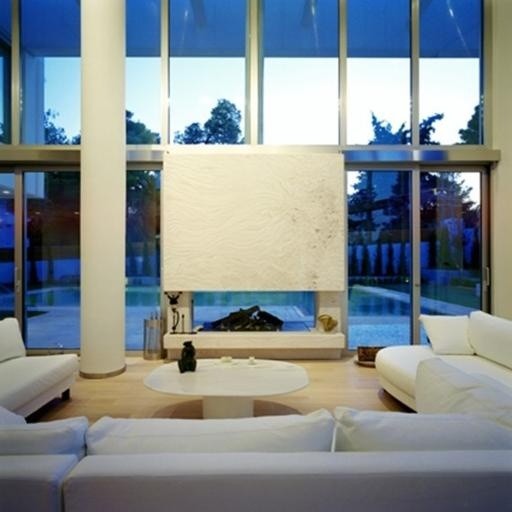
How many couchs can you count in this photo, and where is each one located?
[0,358,512,512]
[375,311,512,413]
[0,317,78,418]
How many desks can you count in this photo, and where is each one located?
[163,331,346,359]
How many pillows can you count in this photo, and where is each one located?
[418,314,473,354]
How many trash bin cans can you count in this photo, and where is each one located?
[144,318,166,360]
